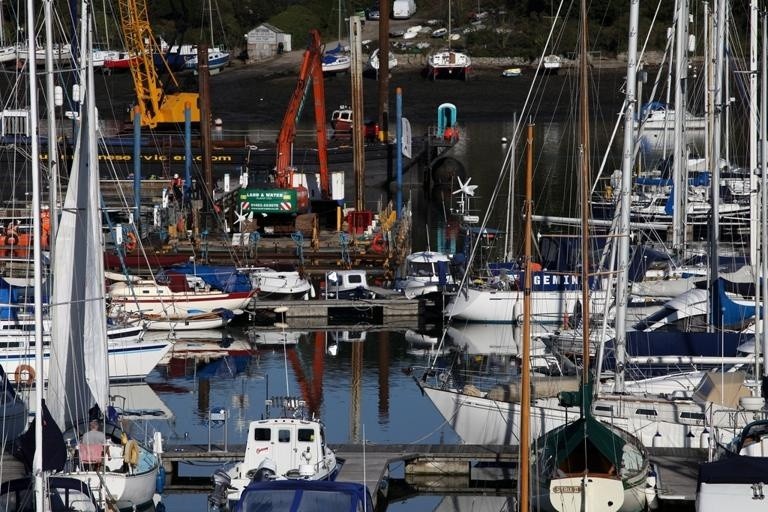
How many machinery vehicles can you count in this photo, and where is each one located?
[238,28,334,237]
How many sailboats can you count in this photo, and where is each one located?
[319,0,353,73]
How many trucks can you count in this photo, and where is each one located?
[393,1,417,19]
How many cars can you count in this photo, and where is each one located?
[355,7,380,20]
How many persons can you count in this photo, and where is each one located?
[81,419,112,471]
[167,172,182,197]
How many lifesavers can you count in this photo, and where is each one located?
[176,217,186,233]
[124,232,137,251]
[124,439,139,468]
[373,234,385,251]
[14,364,36,388]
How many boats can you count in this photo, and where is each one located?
[212,395,342,511]
[368,47,397,71]
[322,270,373,300]
[252,266,311,295]
[331,105,354,130]
